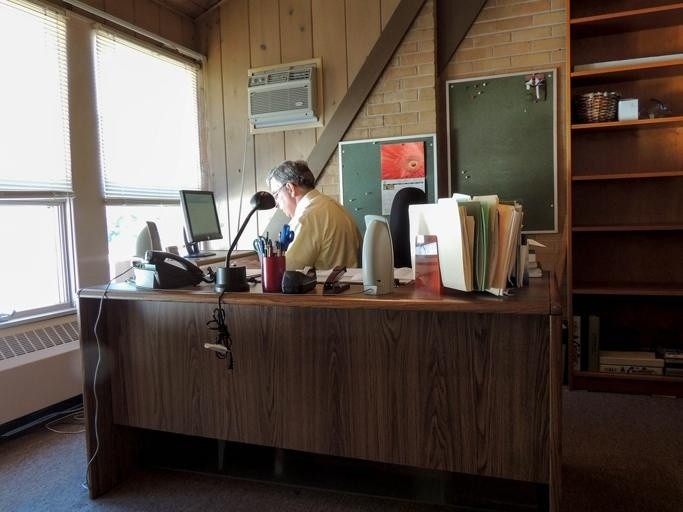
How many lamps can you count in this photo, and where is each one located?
[214,191,276,293]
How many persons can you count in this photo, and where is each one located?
[265,160,363,273]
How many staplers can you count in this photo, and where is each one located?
[323,266,350,294]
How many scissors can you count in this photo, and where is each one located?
[281,224,294,256]
[253,237,266,251]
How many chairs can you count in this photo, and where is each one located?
[387,186,428,269]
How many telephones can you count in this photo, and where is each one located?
[131,250,204,289]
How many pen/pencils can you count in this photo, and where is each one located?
[258,231,285,257]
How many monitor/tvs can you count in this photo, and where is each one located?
[179,189,224,258]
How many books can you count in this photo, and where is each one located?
[571,313,683,375]
[407,192,531,298]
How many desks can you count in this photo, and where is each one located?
[74,250,564,512]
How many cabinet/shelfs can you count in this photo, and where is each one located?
[564,0,683,397]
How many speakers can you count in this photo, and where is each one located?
[134,221,161,259]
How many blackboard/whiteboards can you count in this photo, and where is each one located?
[338,133,438,268]
[446,67,559,234]
